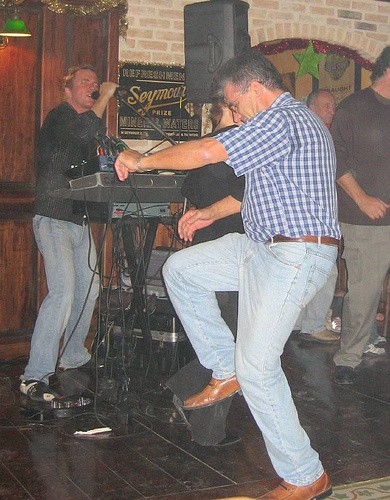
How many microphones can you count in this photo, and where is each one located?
[91,87,124,99]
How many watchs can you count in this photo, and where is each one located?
[137,153,153,171]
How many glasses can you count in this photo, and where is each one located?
[229,90,247,113]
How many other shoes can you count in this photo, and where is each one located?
[363,335,387,357]
[199,436,241,446]
[19,377,55,402]
[334,365,358,385]
[330,316,342,333]
[162,382,191,423]
[300,329,341,343]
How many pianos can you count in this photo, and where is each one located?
[65,149,188,403]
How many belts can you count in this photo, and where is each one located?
[266,233,340,246]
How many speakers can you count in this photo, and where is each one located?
[183,0,252,103]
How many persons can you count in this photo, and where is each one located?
[293,89,342,344]
[324,296,343,333]
[20,64,120,398]
[332,46,390,384]
[114,52,342,500]
[165,79,245,446]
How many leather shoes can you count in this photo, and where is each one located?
[182,376,243,410]
[255,470,332,500]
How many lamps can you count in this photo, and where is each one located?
[0,0,32,49]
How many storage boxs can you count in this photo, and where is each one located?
[99,247,196,376]
